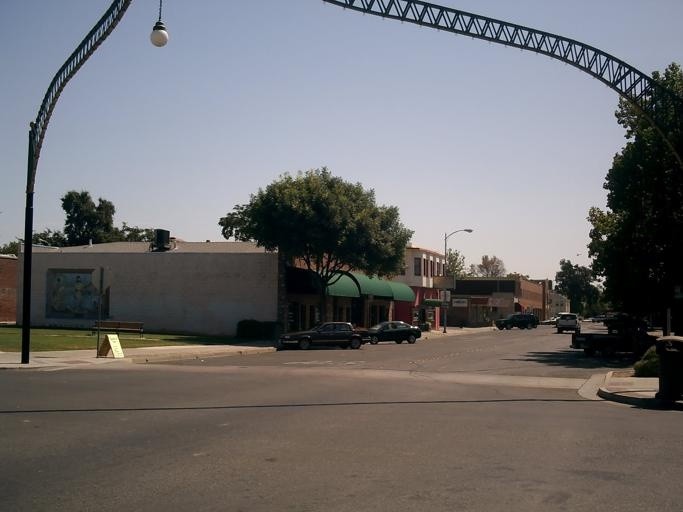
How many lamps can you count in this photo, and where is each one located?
[151,0,169,47]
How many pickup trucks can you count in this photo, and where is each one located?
[570,319,657,355]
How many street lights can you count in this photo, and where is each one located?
[444,228,473,333]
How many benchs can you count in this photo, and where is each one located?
[92,321,144,338]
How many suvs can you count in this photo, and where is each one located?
[557,313,580,334]
[496,314,540,329]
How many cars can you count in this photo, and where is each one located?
[362,321,421,344]
[278,322,363,350]
